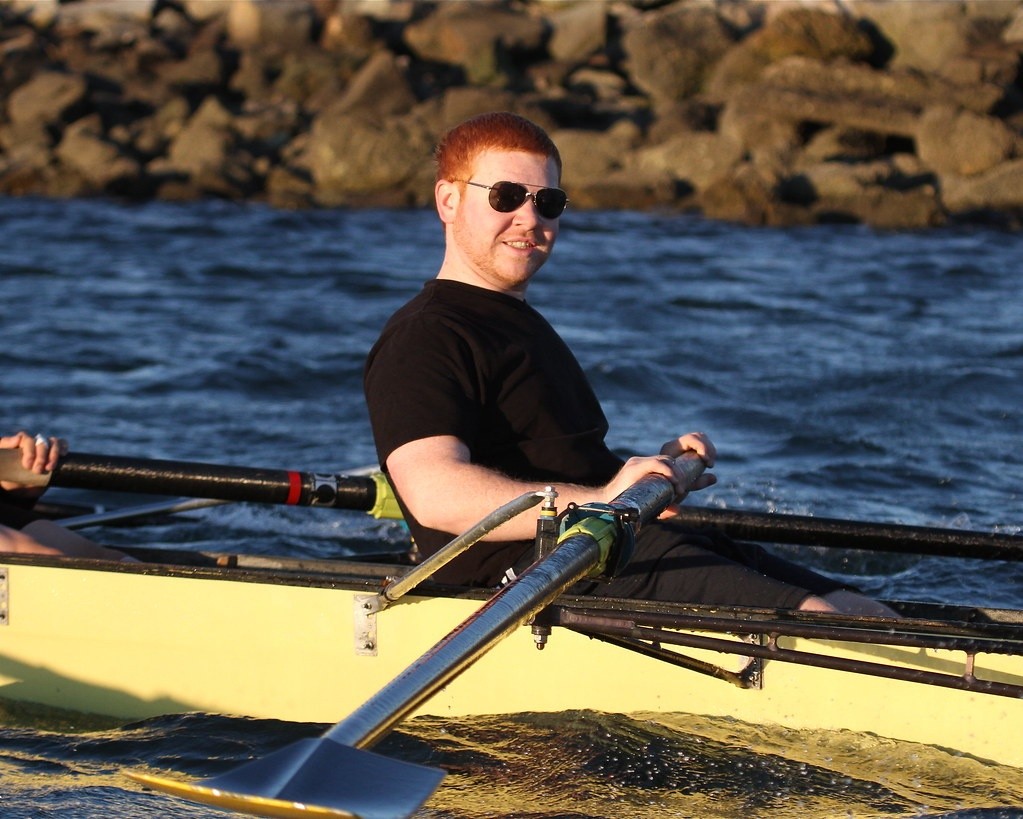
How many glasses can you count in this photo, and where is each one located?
[467,180,570,220]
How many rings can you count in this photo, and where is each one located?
[35,435,49,447]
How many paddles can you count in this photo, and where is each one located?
[0,446,1023,563]
[119,448,706,819]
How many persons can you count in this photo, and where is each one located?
[1,431,142,563]
[364,112,899,619]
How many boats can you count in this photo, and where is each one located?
[0,548,1022,775]
